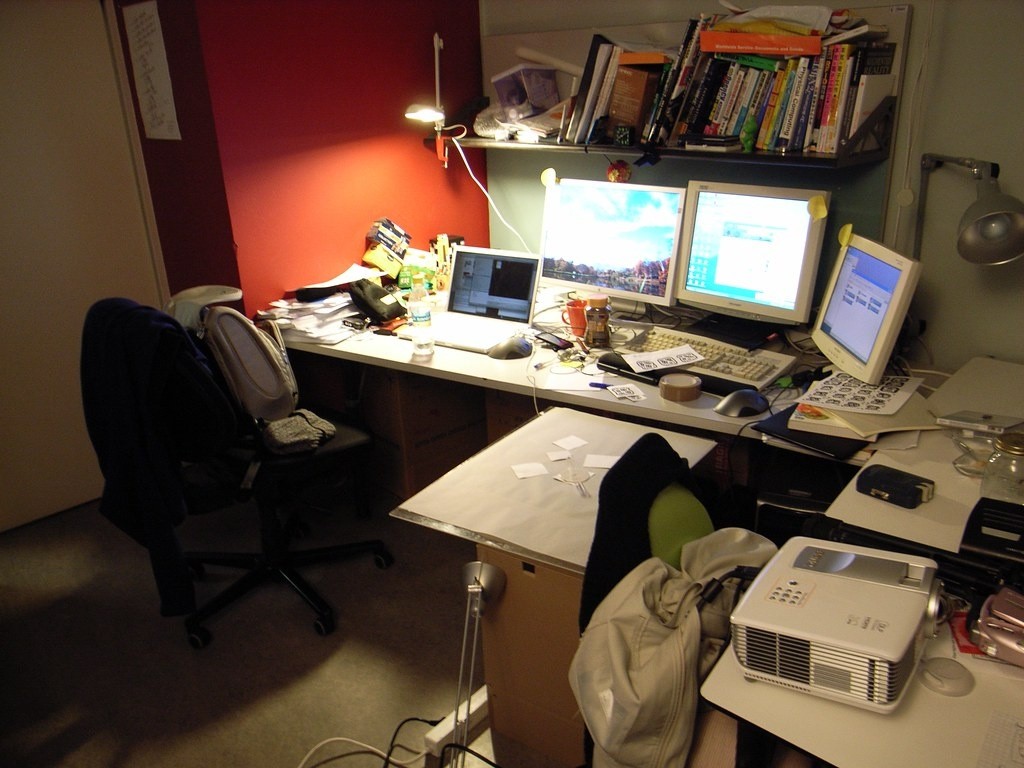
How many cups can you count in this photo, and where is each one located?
[562,300,586,336]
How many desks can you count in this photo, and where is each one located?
[277,277,1024,768]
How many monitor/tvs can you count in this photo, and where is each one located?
[672,180,833,350]
[810,232,922,387]
[538,179,687,326]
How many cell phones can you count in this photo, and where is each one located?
[534,332,574,350]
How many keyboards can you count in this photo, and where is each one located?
[614,325,798,393]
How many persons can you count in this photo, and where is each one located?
[544,255,672,296]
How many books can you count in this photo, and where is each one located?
[936,409,1024,435]
[516,14,896,154]
[751,381,954,459]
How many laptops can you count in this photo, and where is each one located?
[398,244,545,353]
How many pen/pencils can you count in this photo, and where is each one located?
[576,338,589,355]
[589,383,612,389]
[534,355,560,369]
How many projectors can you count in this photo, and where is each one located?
[728,536,944,716]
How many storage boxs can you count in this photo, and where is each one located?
[491,61,560,123]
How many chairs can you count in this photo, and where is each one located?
[90,298,394,649]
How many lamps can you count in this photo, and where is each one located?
[403,104,446,132]
[899,153,1024,354]
[449,560,507,768]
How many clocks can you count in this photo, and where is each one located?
[613,124,635,146]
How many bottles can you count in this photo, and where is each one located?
[584,295,610,348]
[981,433,1024,504]
[407,275,434,355]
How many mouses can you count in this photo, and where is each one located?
[488,336,531,360]
[713,388,769,417]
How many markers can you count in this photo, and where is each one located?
[748,332,779,353]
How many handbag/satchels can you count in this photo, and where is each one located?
[568,524,780,768]
[159,277,298,424]
